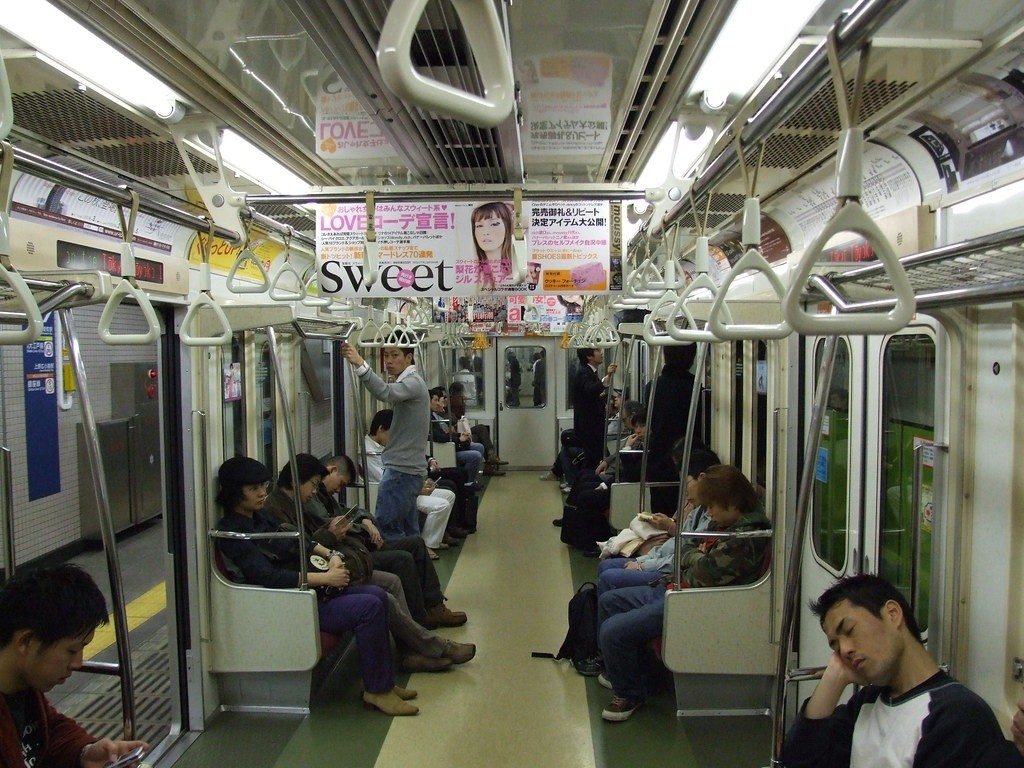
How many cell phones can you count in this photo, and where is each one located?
[431,476,441,486]
[103,746,146,768]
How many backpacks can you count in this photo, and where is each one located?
[316,515,373,586]
[532,582,599,660]
[267,523,339,598]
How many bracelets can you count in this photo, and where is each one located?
[325,549,345,561]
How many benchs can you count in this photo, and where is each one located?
[603,480,652,536]
[651,566,776,715]
[208,556,340,716]
[349,480,378,517]
[425,440,457,469]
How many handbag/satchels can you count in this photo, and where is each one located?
[561,511,609,547]
[457,416,471,436]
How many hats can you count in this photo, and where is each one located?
[219,456,271,486]
[614,387,630,401]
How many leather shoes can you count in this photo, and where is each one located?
[483,467,506,476]
[362,685,419,715]
[487,454,509,465]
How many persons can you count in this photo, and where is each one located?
[567,435,773,723]
[557,295,586,315]
[433,298,506,322]
[778,571,1024,768]
[830,387,848,412]
[643,341,710,518]
[301,452,467,631]
[225,363,237,399]
[1010,698,1024,759]
[258,452,477,671]
[340,328,655,561]
[213,455,419,717]
[471,201,527,293]
[1,560,151,768]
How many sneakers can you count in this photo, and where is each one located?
[412,603,467,630]
[427,636,476,664]
[597,673,614,691]
[539,470,565,481]
[601,693,640,722]
[397,655,452,672]
[575,653,604,676]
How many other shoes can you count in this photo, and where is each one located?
[583,550,600,558]
[442,529,476,546]
[427,548,440,560]
[559,482,572,494]
[553,519,562,527]
[471,482,485,491]
[431,543,449,550]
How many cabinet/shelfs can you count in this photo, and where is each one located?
[76,415,137,541]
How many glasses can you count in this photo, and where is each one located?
[249,481,269,494]
[309,479,320,492]
[622,413,632,422]
